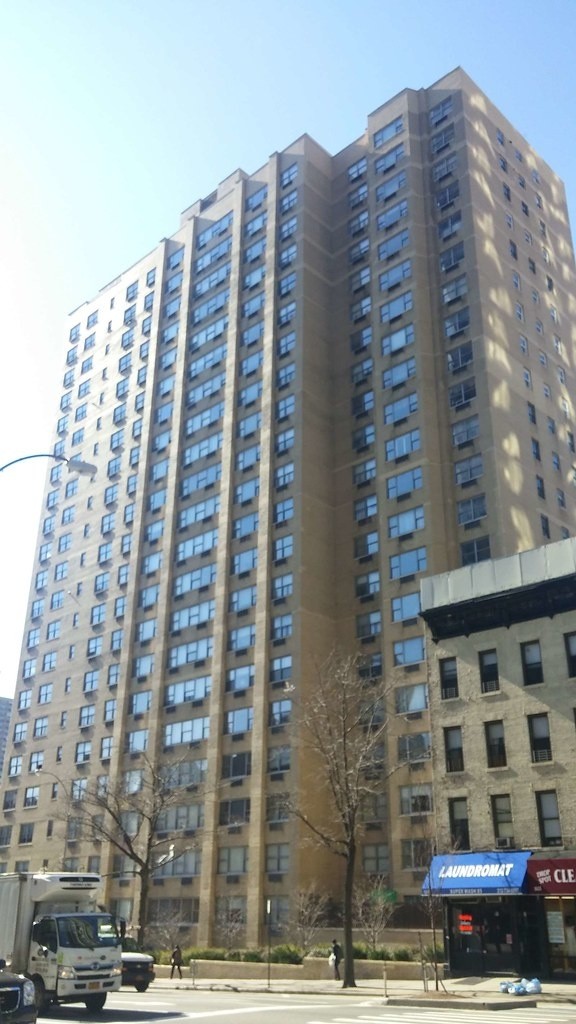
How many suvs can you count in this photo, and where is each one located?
[96,933,155,992]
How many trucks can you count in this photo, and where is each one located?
[0,872,122,1017]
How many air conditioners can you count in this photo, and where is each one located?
[495,838,513,849]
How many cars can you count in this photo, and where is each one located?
[0,959,37,1024]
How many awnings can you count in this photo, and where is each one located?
[415,852,532,899]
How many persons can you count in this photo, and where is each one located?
[169,944,183,980]
[332,938,342,981]
